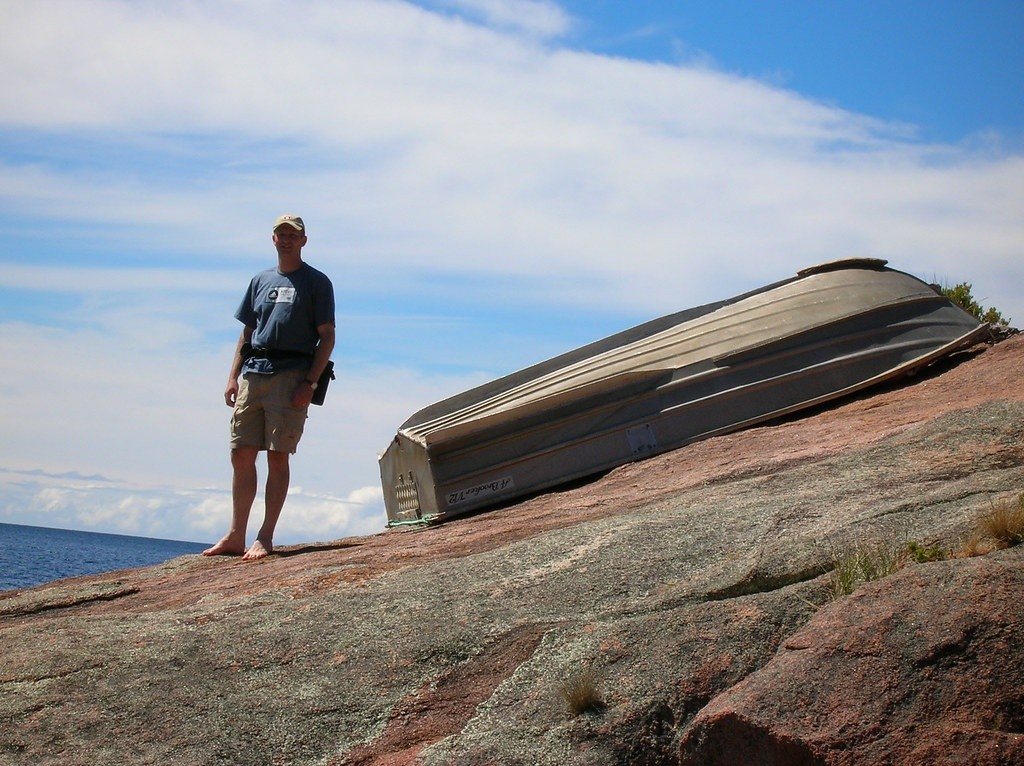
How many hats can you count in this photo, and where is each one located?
[272,215,305,236]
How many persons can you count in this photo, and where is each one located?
[202,214,336,559]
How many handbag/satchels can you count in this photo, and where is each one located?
[310,360,336,406]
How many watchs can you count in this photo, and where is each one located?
[303,379,318,390]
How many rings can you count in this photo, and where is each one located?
[302,404,306,407]
